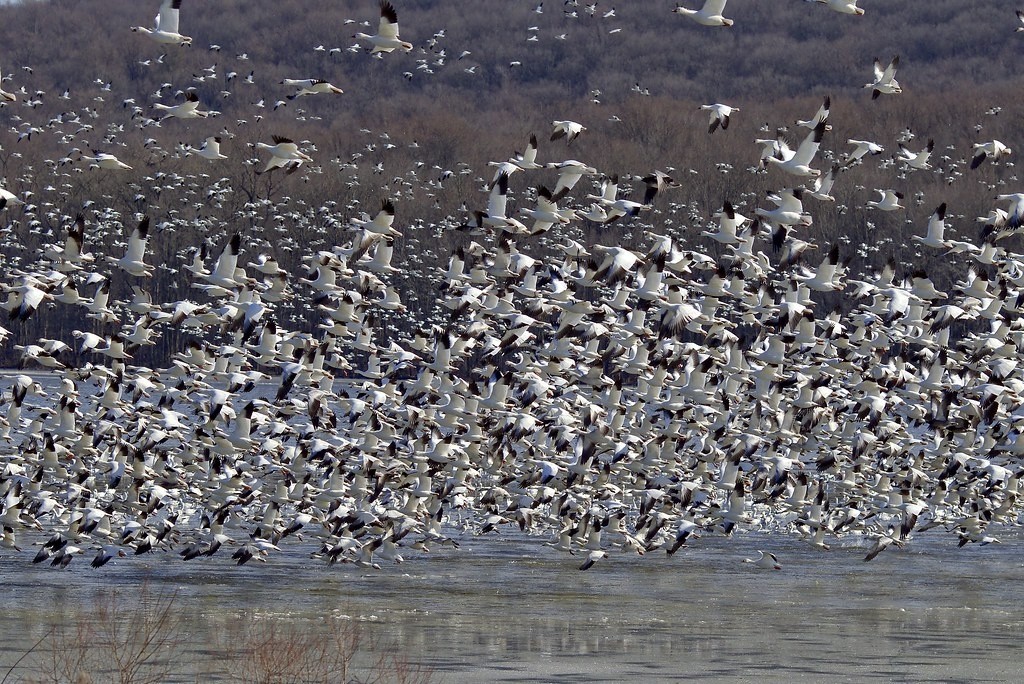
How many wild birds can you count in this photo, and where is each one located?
[2,0,1024,583]
